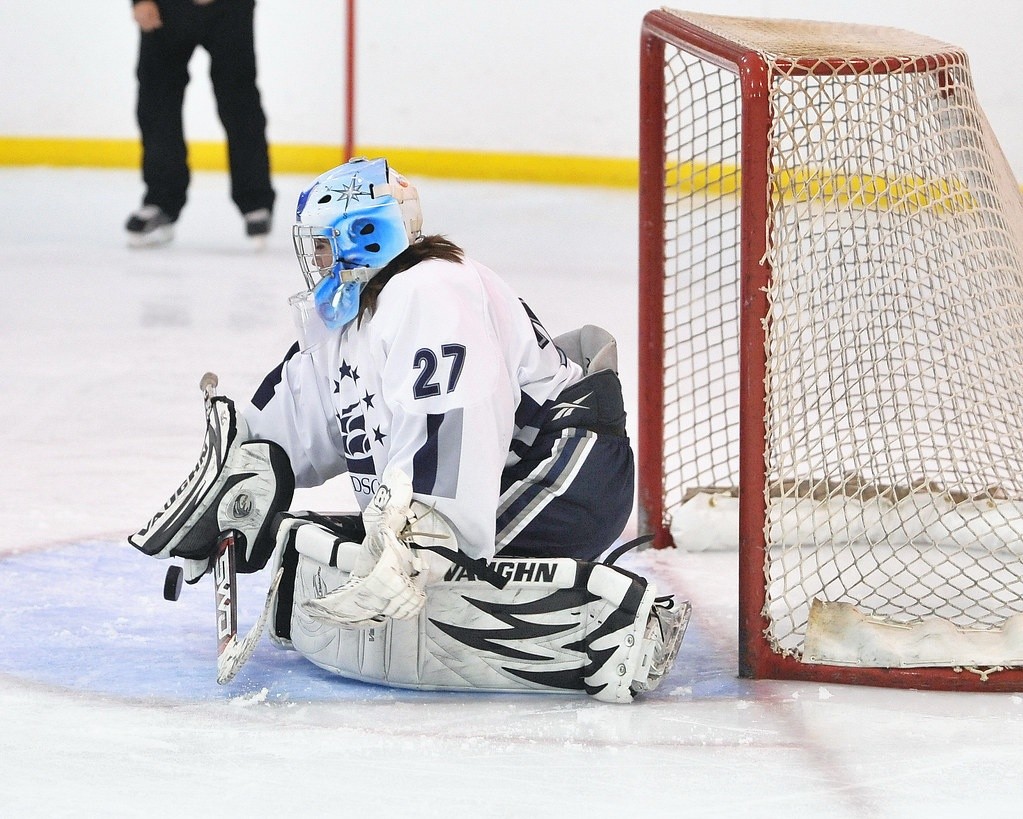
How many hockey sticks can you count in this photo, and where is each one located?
[199,372,285,686]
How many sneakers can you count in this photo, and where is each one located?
[630,594,692,693]
[243,207,274,250]
[124,204,176,246]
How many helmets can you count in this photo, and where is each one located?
[293,156,423,329]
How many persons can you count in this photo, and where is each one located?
[128,159,689,707]
[124,0,276,253]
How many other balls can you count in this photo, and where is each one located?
[164,566,183,602]
